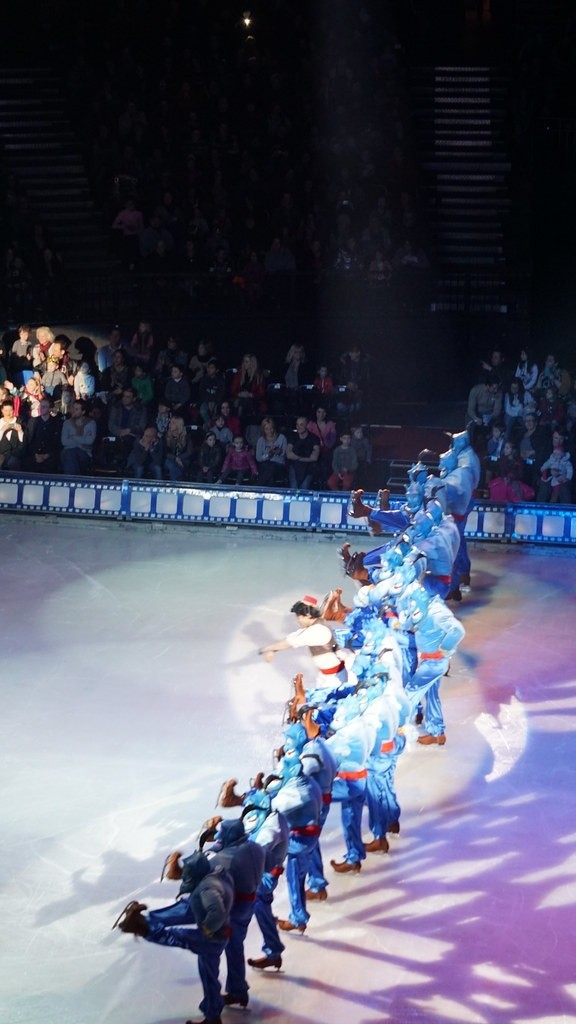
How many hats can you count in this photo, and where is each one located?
[299,595,318,606]
[553,445,565,456]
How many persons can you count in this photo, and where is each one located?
[0,0,433,314]
[118,430,483,1023]
[463,333,575,504]
[509,0,576,161]
[0,322,373,492]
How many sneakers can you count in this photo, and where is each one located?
[112,900,150,938]
[374,490,390,512]
[195,817,221,844]
[290,673,305,704]
[367,518,381,537]
[345,489,371,518]
[346,552,369,581]
[459,575,472,594]
[299,706,319,740]
[331,860,362,876]
[319,590,346,622]
[187,1018,225,1024]
[215,780,244,806]
[444,589,462,602]
[219,992,251,1012]
[274,745,285,770]
[160,852,184,882]
[281,696,299,726]
[362,840,389,855]
[303,889,327,903]
[417,733,447,748]
[277,919,308,938]
[333,587,352,612]
[248,956,285,974]
[250,773,263,790]
[385,822,400,837]
[337,543,350,569]
